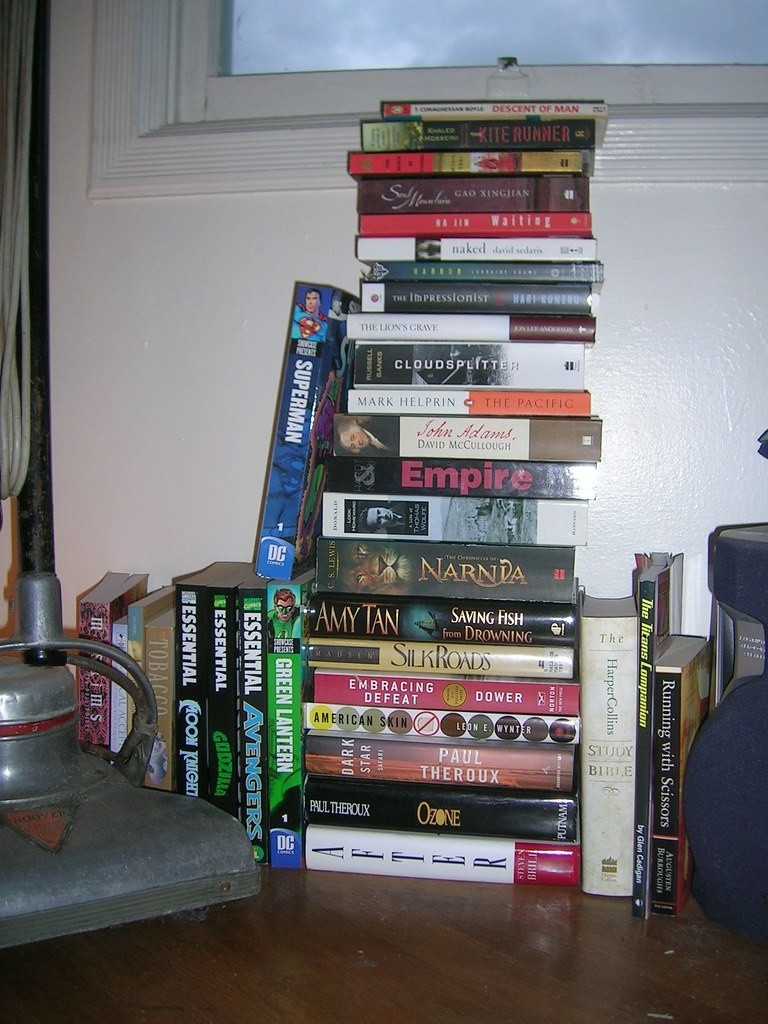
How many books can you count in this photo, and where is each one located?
[76,103,610,886]
[578,552,708,919]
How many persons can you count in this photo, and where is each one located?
[360,503,407,534]
[340,419,399,454]
